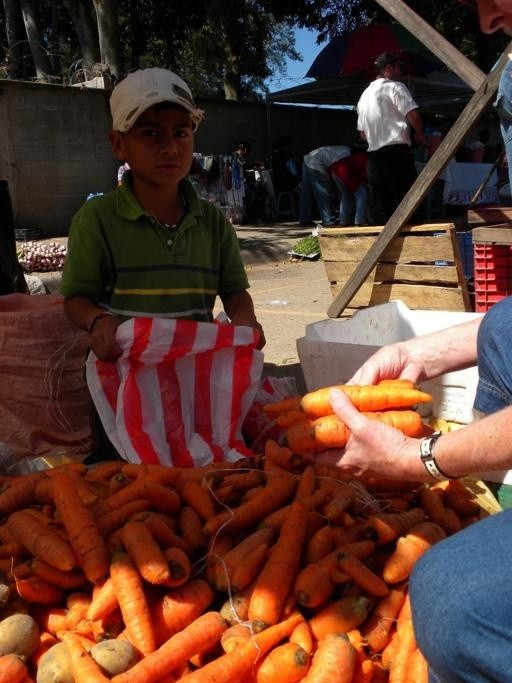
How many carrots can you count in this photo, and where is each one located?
[1,376,489,681]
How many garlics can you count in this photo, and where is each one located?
[17,240,67,271]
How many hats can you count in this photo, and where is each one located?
[374,51,399,68]
[108,66,204,134]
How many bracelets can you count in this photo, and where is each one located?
[86,314,111,336]
[421,430,459,481]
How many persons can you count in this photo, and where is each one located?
[61,68,265,465]
[233,51,428,225]
[308,1,511,683]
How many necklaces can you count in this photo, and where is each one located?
[160,195,188,232]
